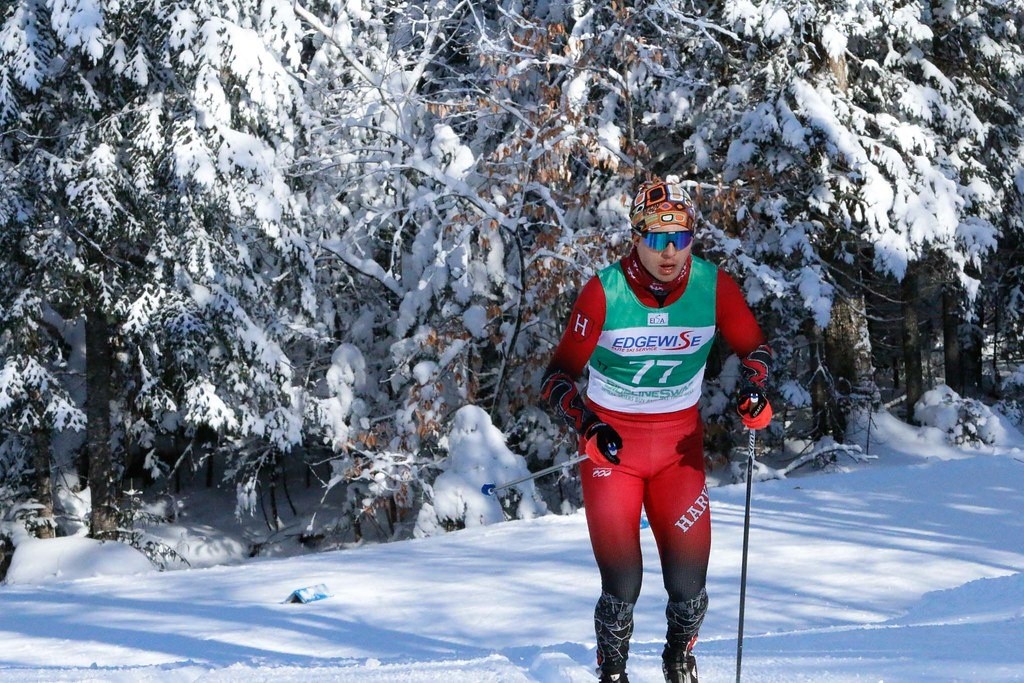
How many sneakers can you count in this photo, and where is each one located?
[599,665,629,683]
[662,647,698,683]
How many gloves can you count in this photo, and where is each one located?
[585,423,622,467]
[737,382,772,430]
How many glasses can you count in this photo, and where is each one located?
[642,230,695,251]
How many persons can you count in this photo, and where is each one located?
[537,181,772,683]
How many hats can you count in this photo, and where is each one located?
[629,181,696,243]
